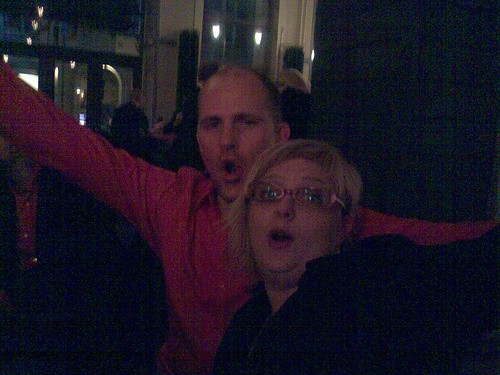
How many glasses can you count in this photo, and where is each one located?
[245,179,351,220]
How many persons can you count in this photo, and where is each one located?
[268,45,314,137]
[167,61,223,176]
[0,142,86,375]
[1,57,499,375]
[208,137,438,375]
[110,86,153,156]
